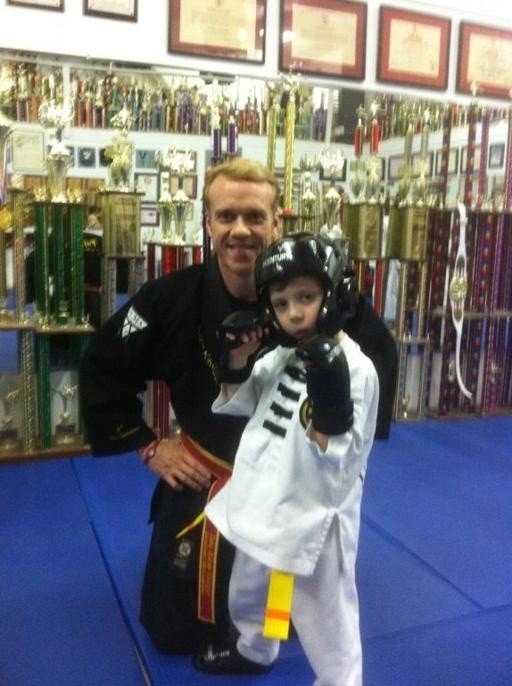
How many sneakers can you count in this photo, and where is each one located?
[193,641,274,676]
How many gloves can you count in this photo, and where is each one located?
[214,307,272,382]
[293,332,355,435]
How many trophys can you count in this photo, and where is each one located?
[0,48,435,452]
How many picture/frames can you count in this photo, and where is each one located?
[7,0,66,15]
[135,173,196,225]
[375,3,451,91]
[279,1,367,80]
[168,0,266,64]
[456,21,512,99]
[82,0,138,22]
[374,141,511,181]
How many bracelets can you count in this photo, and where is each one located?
[143,440,162,465]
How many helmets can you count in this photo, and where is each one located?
[252,226,360,339]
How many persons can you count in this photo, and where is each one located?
[74,159,398,658]
[192,229,382,686]
